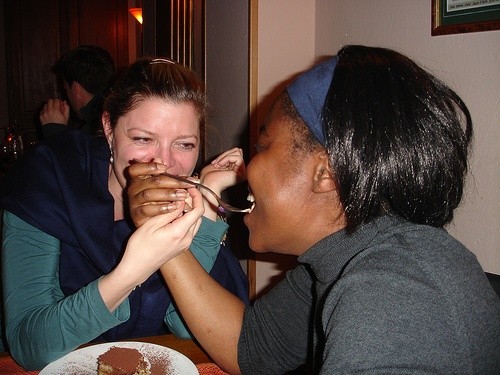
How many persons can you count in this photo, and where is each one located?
[0,54,250,371]
[127,44,500,375]
[38,44,115,144]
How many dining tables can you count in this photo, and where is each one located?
[1,334,214,375]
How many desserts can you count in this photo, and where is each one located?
[96,346,150,375]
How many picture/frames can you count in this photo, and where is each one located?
[431,0,500,36]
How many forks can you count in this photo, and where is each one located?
[128,159,250,213]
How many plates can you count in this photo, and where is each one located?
[39,341,198,374]
